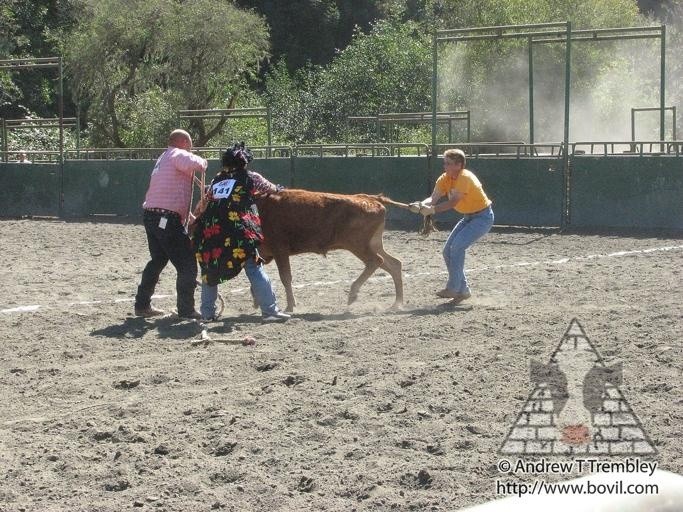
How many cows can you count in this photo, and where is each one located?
[188,170,438,312]
[525,349,623,445]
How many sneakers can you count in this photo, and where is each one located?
[178,310,201,321]
[262,310,291,322]
[436,287,471,305]
[201,311,219,323]
[134,308,165,316]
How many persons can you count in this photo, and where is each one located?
[187,141,291,324]
[134,127,208,322]
[406,148,497,307]
[15,150,31,163]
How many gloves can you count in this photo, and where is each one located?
[409,201,435,216]
